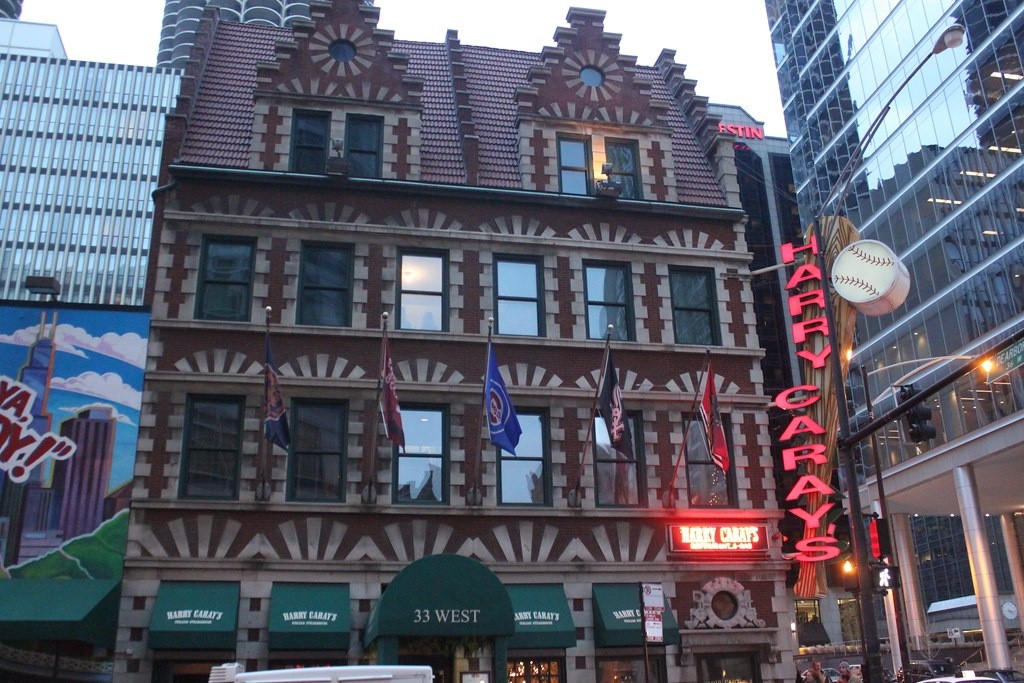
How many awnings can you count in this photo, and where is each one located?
[266,582,351,651]
[592,583,680,648]
[148,580,239,651]
[359,553,515,683]
[0,578,121,652]
[504,583,576,649]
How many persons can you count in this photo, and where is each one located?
[803,660,832,683]
[836,660,862,683]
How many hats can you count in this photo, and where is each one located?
[839,660,851,672]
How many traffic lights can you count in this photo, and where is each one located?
[841,555,859,592]
[871,565,900,588]
[892,385,936,443]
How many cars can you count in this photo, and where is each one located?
[801,664,863,683]
[883,660,1024,683]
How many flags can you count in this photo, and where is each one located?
[263,343,292,451]
[598,353,634,459]
[483,344,522,455]
[700,363,729,473]
[380,333,405,447]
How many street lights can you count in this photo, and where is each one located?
[862,355,994,683]
[813,23,966,683]
